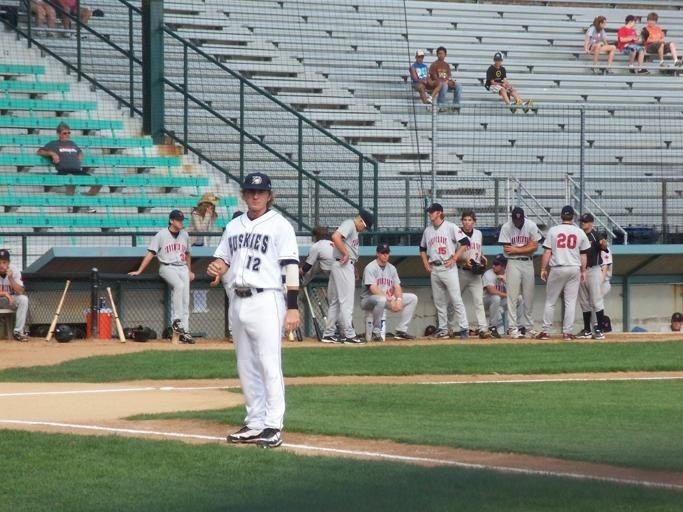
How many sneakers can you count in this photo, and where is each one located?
[661,59,683,72]
[372,332,383,342]
[172,319,185,335]
[510,100,520,113]
[37,28,89,39]
[628,65,648,73]
[563,326,605,341]
[14,334,29,342]
[427,326,550,340]
[321,336,365,345]
[256,428,283,448]
[394,331,417,341]
[179,334,195,344]
[523,99,532,113]
[592,68,613,75]
[425,98,459,113]
[228,426,264,443]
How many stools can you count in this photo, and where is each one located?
[0,308,17,342]
[485,305,504,329]
[365,307,386,343]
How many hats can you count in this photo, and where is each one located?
[512,208,524,219]
[424,203,443,212]
[198,193,220,206]
[0,250,9,259]
[494,53,502,61]
[169,210,188,221]
[562,206,573,215]
[240,173,271,190]
[359,211,372,233]
[377,242,389,251]
[581,214,594,222]
[672,313,682,322]
[494,254,505,265]
[416,51,424,57]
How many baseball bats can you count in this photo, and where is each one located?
[106,287,127,343]
[301,278,322,341]
[283,283,302,341]
[45,280,71,342]
[312,287,340,336]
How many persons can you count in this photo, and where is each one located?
[663,312,683,332]
[0,249,29,341]
[300,213,418,343]
[128,209,196,343]
[419,203,611,340]
[38,122,102,213]
[22,1,91,38]
[190,193,217,246]
[207,172,301,448]
[410,47,460,112]
[486,53,533,113]
[586,12,683,74]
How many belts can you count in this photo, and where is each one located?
[434,262,441,266]
[336,259,353,264]
[235,288,263,297]
[511,258,532,261]
